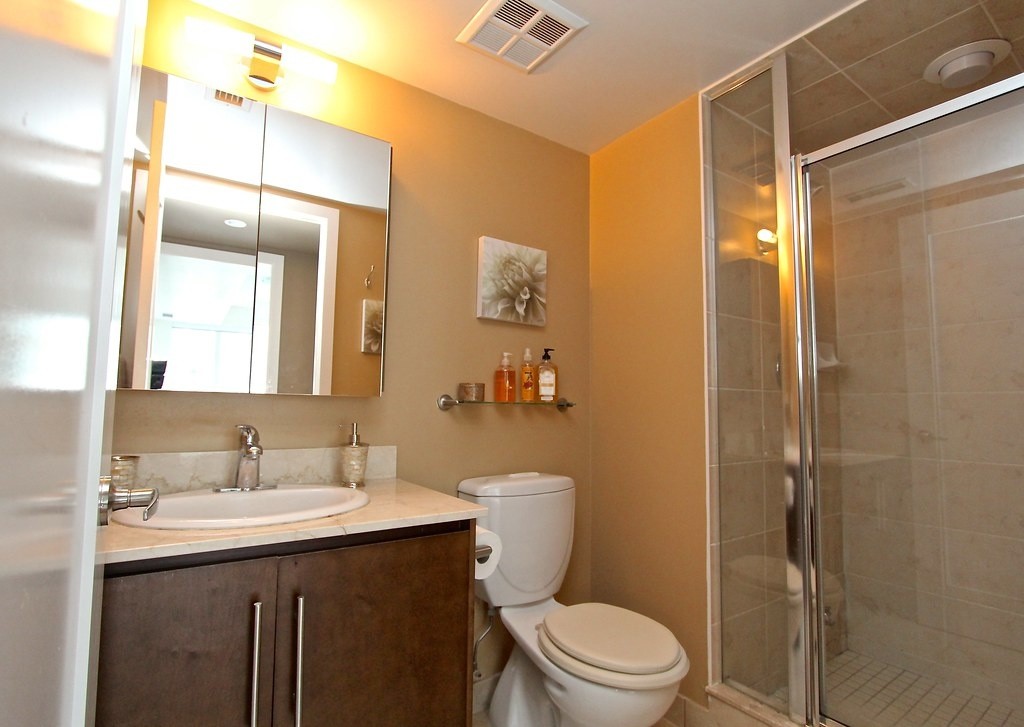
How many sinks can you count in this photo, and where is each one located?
[110,482,371,528]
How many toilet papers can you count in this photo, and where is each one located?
[475,523,504,580]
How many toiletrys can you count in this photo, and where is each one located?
[456,346,561,402]
[338,421,370,488]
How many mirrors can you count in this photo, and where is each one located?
[115,65,393,397]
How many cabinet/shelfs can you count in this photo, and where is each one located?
[93,518,476,727]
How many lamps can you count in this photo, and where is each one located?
[248,37,283,91]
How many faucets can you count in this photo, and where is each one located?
[233,423,263,488]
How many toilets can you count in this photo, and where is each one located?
[457,470,691,727]
[717,443,844,649]
